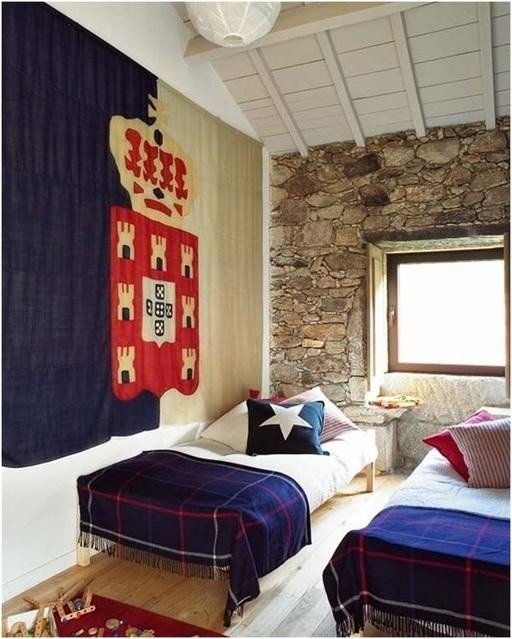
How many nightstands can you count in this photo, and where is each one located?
[354,420,398,472]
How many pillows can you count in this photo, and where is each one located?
[200,399,249,453]
[277,386,360,445]
[446,417,511,488]
[246,398,330,456]
[422,409,497,483]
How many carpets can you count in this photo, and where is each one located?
[52,591,231,637]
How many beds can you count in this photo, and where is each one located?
[77,429,376,624]
[322,447,510,637]
